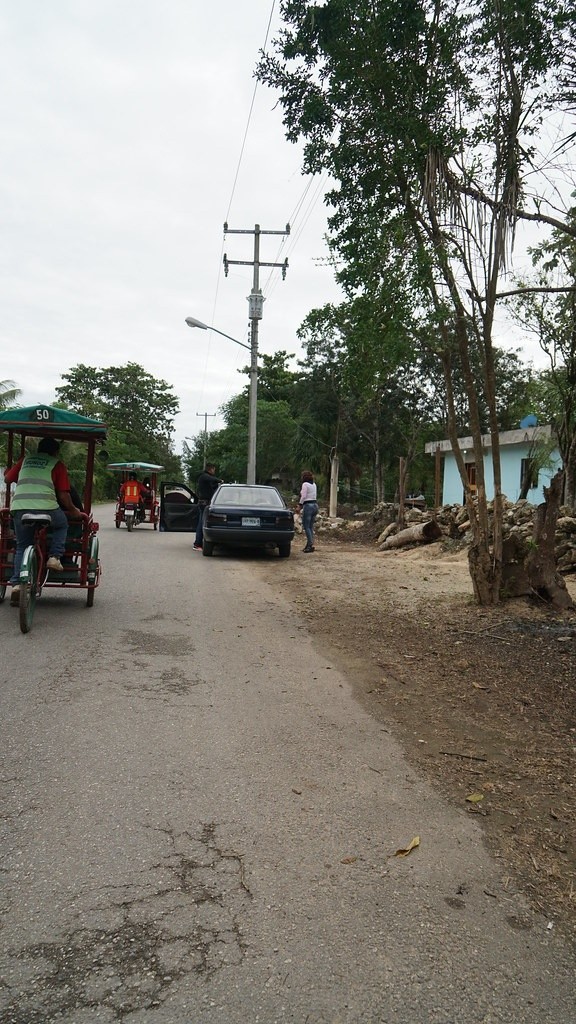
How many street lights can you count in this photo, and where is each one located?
[184,317,261,484]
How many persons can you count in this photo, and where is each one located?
[4,438,92,607]
[193,463,223,550]
[296,471,318,552]
[120,472,151,521]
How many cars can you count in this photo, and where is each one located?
[159,481,295,560]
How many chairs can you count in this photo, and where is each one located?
[51,483,84,546]
[256,498,267,505]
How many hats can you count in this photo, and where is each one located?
[37,436,60,454]
[129,472,137,478]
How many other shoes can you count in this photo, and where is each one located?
[62,557,79,571]
[303,545,315,552]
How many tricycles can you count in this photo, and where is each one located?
[1,402,101,634]
[106,461,168,534]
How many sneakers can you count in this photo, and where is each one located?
[10,585,20,607]
[46,557,64,572]
[193,543,203,551]
